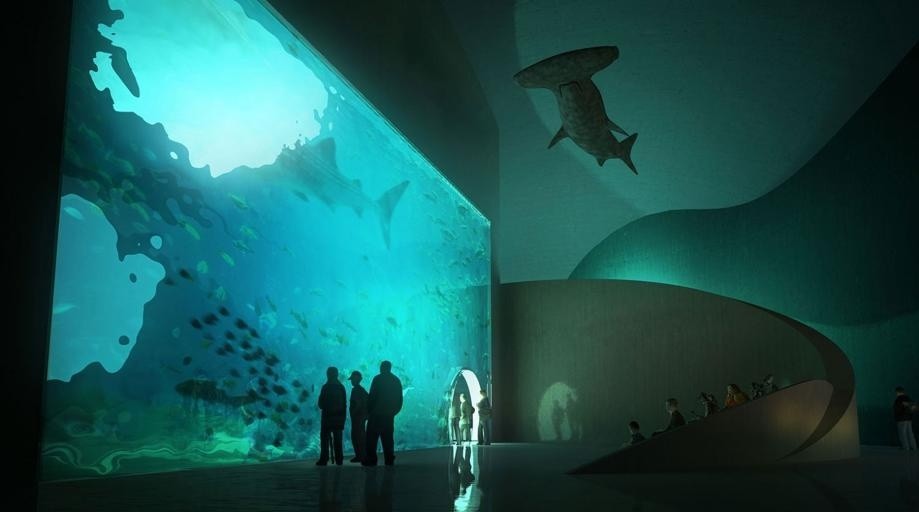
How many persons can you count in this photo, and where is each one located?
[451,389,494,446]
[892,386,918,459]
[313,360,404,469]
[628,383,747,446]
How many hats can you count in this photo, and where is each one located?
[348,370,362,380]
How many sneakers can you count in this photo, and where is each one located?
[315,456,395,467]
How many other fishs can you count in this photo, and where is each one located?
[163,134,492,463]
